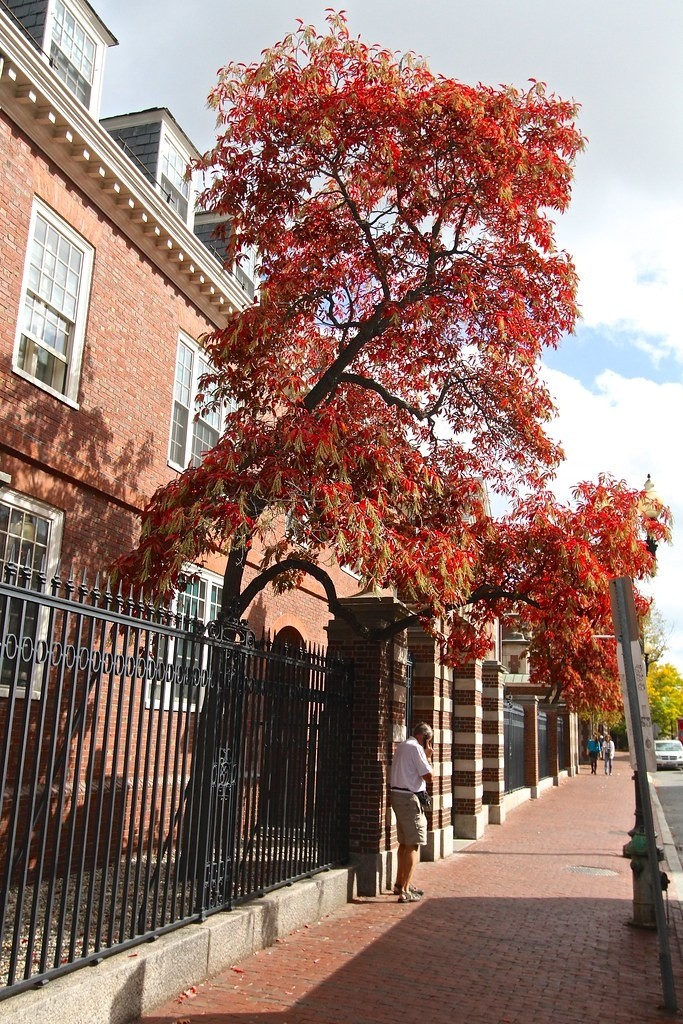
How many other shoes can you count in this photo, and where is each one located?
[591,770,596,775]
[605,772,612,776]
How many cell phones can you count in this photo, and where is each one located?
[423,735,428,749]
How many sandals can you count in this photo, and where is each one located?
[393,884,424,903]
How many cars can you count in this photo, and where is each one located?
[655,740,683,770]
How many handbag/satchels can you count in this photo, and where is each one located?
[415,790,431,806]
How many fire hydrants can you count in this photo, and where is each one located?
[622,826,671,930]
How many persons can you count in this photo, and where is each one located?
[602,735,615,775]
[586,735,600,775]
[599,735,604,760]
[391,722,433,903]
